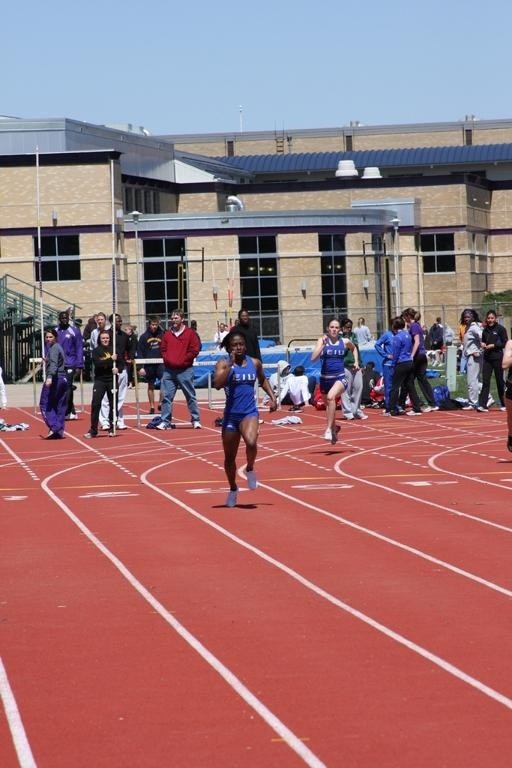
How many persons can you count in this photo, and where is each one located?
[340,317,384,420]
[458,308,512,453]
[312,318,360,443]
[213,329,279,509]
[375,308,454,416]
[214,308,264,425]
[265,360,313,409]
[39,308,202,440]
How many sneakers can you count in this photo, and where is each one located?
[47,406,202,439]
[289,401,440,443]
[226,487,239,508]
[245,470,257,490]
[462,406,512,453]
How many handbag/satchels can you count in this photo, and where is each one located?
[433,385,462,410]
